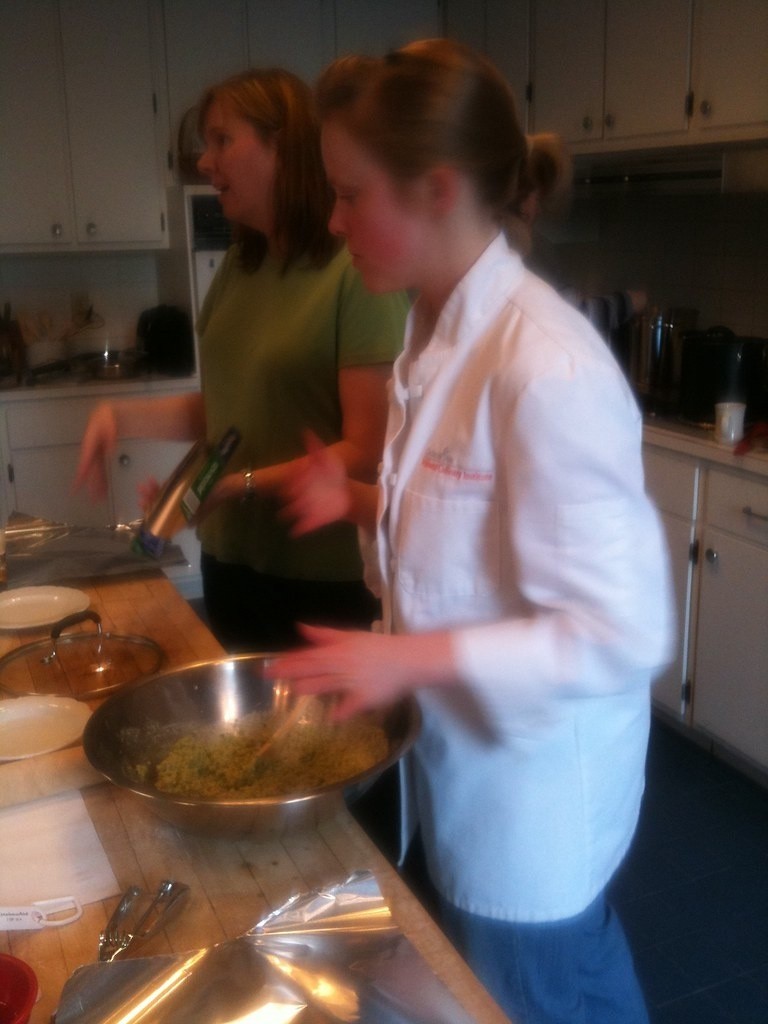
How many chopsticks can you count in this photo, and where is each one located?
[254,694,317,756]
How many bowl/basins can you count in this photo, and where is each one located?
[83,653,423,838]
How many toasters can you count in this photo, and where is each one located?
[134,303,195,379]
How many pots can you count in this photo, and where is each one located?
[680,326,768,423]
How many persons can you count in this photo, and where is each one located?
[70,70,412,849]
[269,40,675,1024]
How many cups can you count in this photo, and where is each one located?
[0,953,38,1024]
[714,402,746,444]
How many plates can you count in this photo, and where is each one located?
[0,694,94,761]
[0,586,90,631]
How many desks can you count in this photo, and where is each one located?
[0,564,510,1024]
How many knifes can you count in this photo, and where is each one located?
[0,302,11,333]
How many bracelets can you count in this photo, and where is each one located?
[242,470,257,507]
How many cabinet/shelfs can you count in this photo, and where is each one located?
[640,430,768,788]
[0,383,204,601]
[0,0,768,255]
